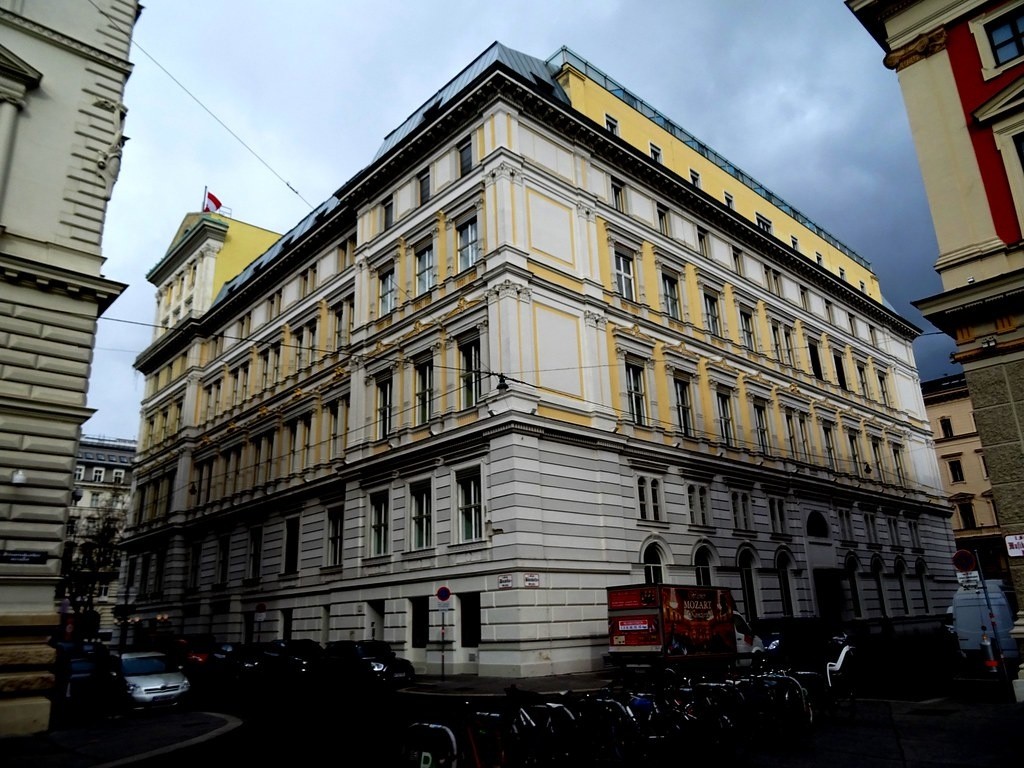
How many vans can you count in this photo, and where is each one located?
[953,580,1020,662]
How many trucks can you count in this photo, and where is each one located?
[605,582,764,682]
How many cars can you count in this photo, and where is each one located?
[178,635,415,691]
[109,650,194,716]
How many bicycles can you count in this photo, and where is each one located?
[451,659,826,768]
